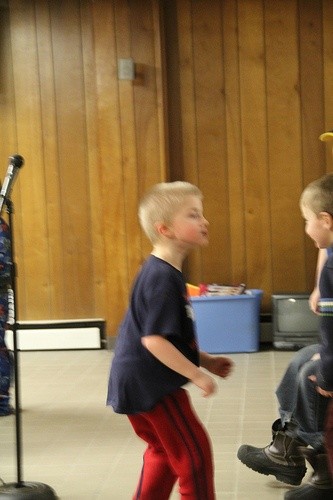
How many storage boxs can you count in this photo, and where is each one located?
[189,287,264,353]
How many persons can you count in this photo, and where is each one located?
[106,178,232,500]
[300,172,332,396]
[236,343,333,500]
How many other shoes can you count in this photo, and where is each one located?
[0,404,11,416]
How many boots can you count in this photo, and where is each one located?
[237,418,308,486]
[283,444,333,500]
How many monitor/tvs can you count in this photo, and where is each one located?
[271,292,325,350]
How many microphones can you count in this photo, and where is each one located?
[0,153,25,215]
